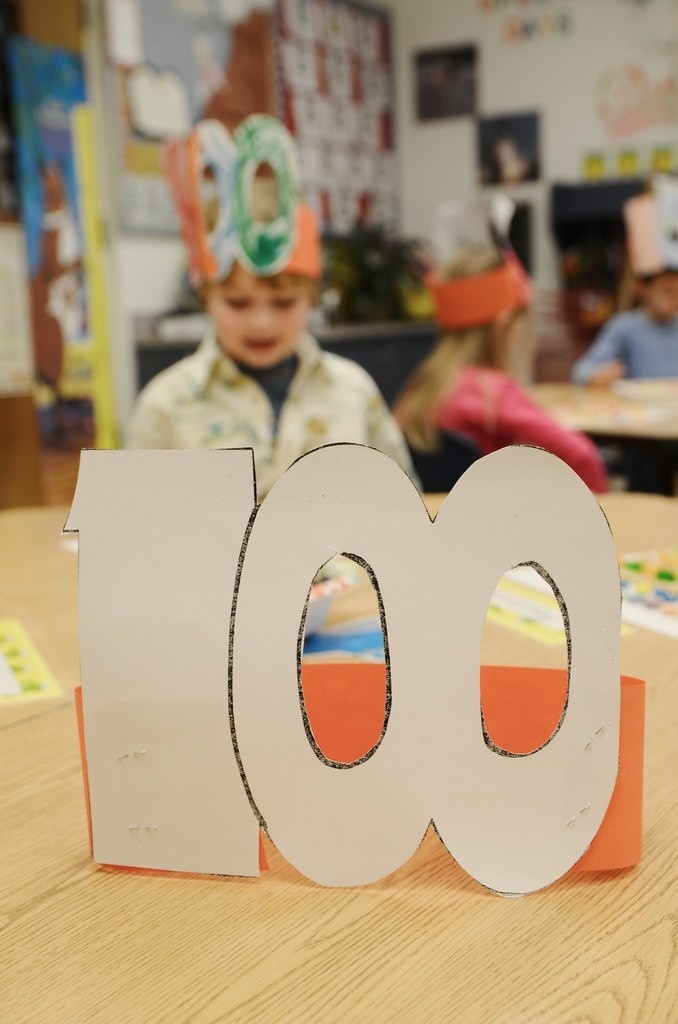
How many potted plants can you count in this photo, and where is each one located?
[320,220,430,324]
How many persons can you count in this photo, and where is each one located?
[562,246,678,502]
[390,237,613,498]
[119,174,425,500]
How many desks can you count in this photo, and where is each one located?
[0,493,678,1024]
[518,375,678,439]
